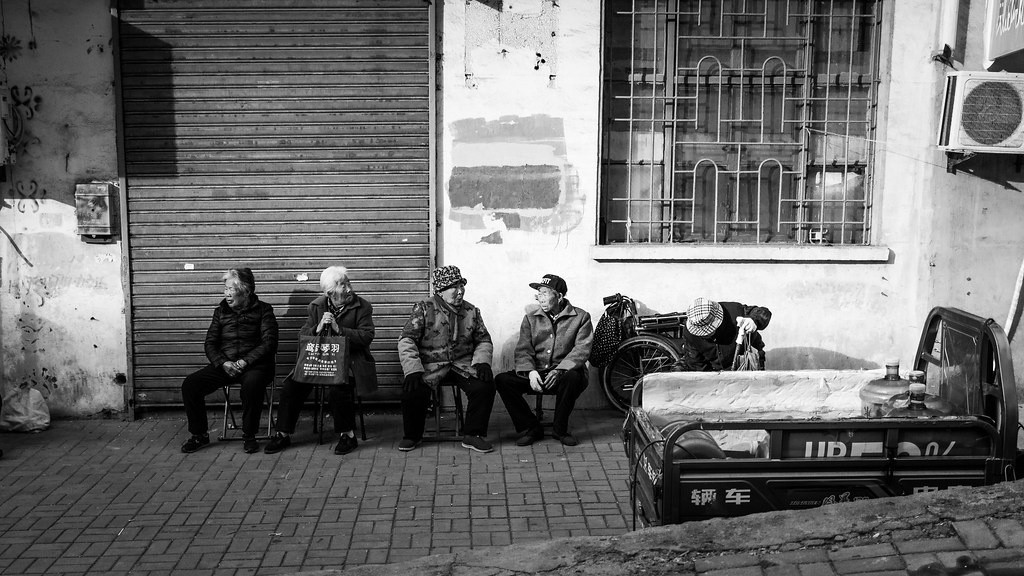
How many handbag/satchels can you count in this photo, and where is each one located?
[588,298,623,367]
[291,322,350,385]
[622,299,637,339]
[0,379,52,432]
[736,329,760,371]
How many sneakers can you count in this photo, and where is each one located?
[551,429,578,446]
[241,433,260,453]
[264,431,290,453]
[335,432,358,454]
[517,423,544,445]
[399,436,422,451]
[461,432,493,452]
[181,433,210,453]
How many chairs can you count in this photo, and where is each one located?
[214,344,279,441]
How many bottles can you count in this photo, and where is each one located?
[858,359,910,419]
[880,370,953,417]
[658,419,726,459]
[882,384,945,418]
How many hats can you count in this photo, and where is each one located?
[432,266,467,293]
[686,297,723,336]
[529,274,568,297]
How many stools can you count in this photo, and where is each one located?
[526,391,556,429]
[312,376,365,444]
[420,371,467,443]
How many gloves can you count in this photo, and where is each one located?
[402,373,424,393]
[473,362,493,383]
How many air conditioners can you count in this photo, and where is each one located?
[934,71,1024,155]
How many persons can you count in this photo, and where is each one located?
[683,297,772,373]
[398,265,495,452]
[181,268,280,453]
[494,274,593,446]
[264,265,377,454]
